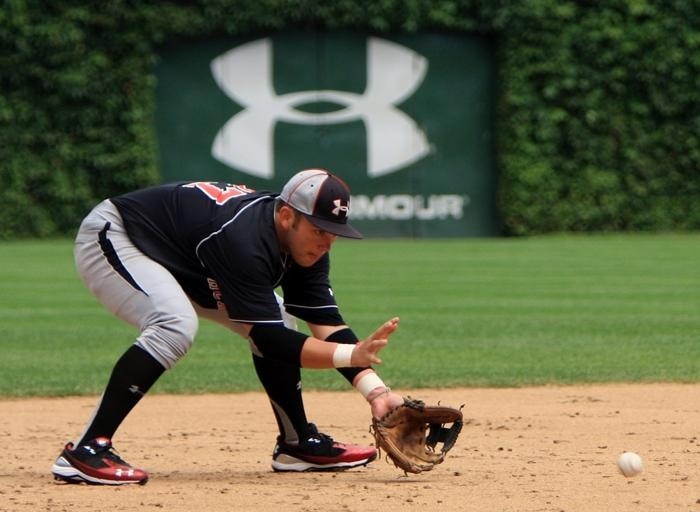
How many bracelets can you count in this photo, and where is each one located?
[356,373,386,396]
[369,387,392,403]
[333,343,355,369]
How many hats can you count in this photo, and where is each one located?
[281,169,362,239]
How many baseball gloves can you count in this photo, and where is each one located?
[371,396,463,474]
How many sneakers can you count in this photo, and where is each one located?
[272,423,377,472]
[51,437,148,485]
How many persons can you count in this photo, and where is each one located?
[50,168,404,486]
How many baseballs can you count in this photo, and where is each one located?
[618,451,642,477]
